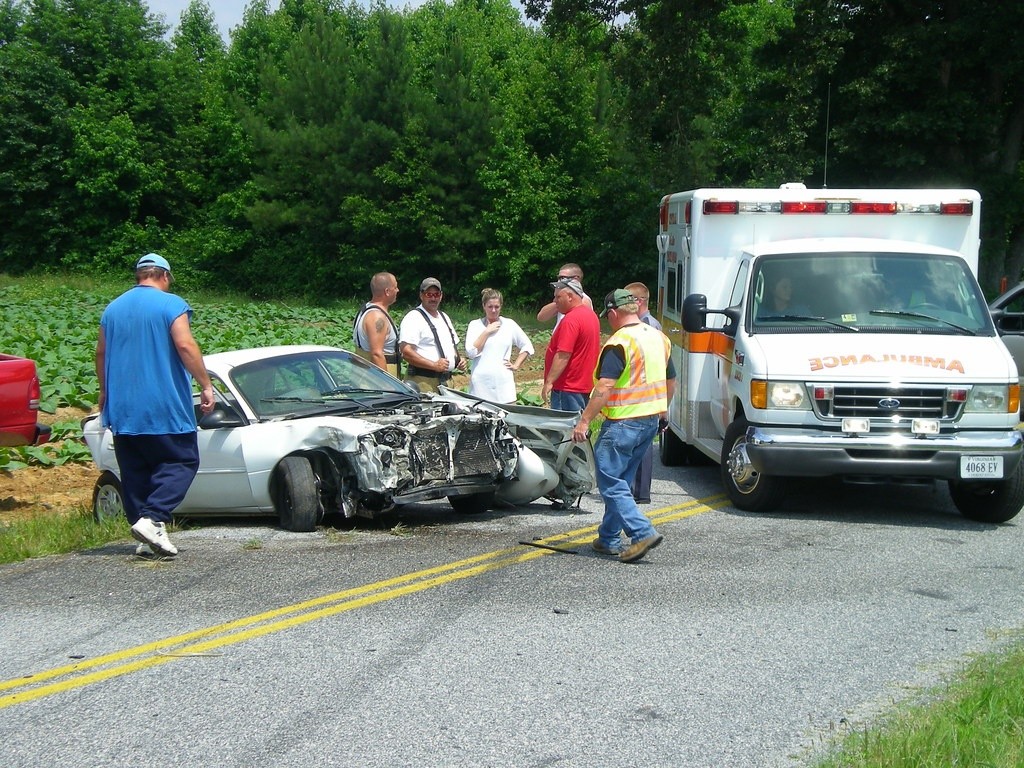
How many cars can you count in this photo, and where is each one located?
[81,343,597,534]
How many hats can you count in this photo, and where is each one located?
[599,289,636,318]
[136,253,176,283]
[421,277,441,294]
[550,279,584,299]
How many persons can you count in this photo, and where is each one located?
[537,263,600,411]
[353,272,402,379]
[572,283,677,564]
[96,253,216,557]
[863,262,948,312]
[756,271,816,317]
[465,288,535,406]
[399,277,467,395]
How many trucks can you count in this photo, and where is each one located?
[658,183,1024,525]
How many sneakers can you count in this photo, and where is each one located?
[130,516,178,557]
[592,537,620,555]
[619,533,663,562]
[134,542,158,557]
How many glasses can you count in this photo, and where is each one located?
[557,275,579,280]
[422,291,441,298]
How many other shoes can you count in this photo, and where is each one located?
[633,496,650,504]
